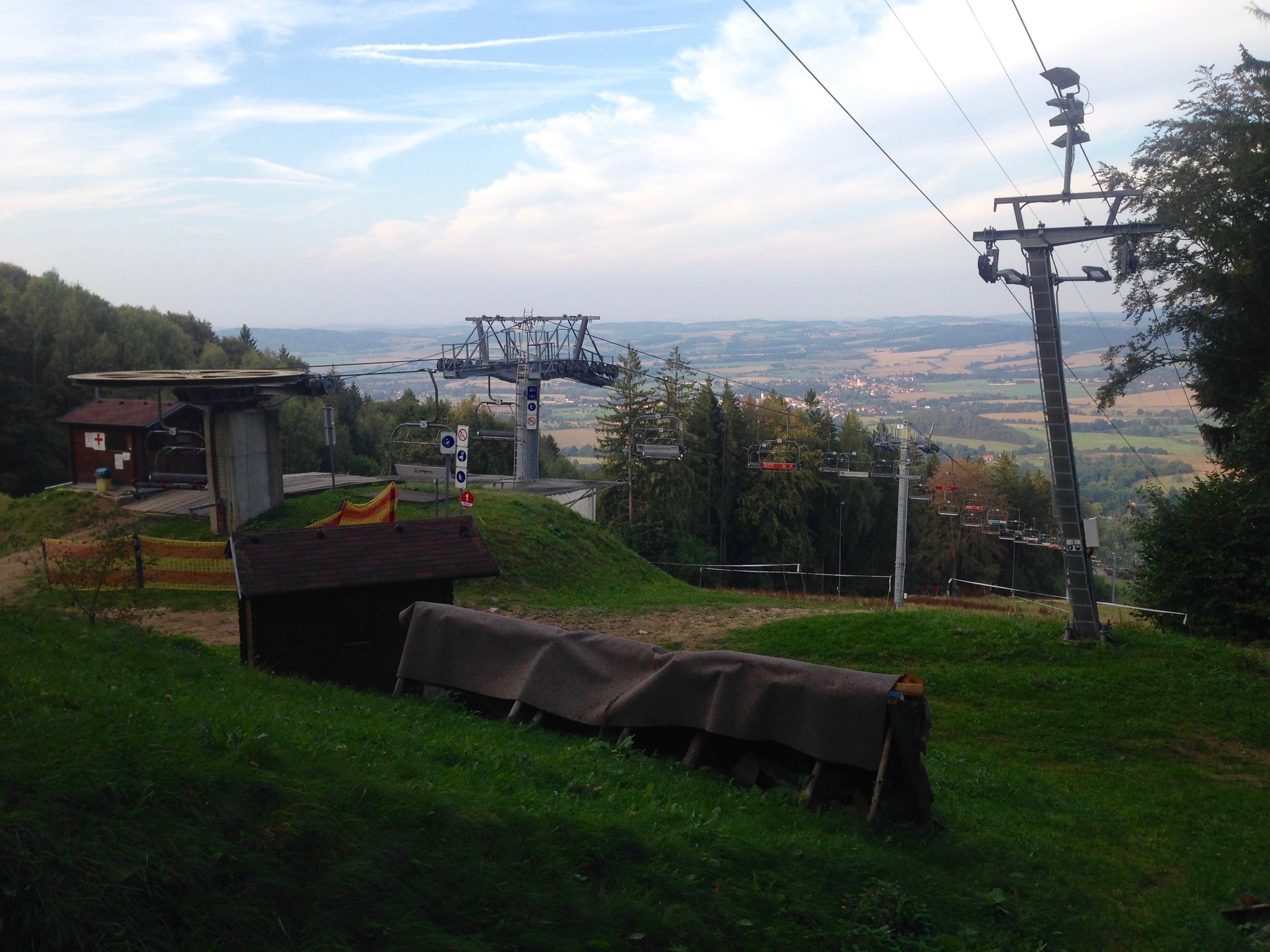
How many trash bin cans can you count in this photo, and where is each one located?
[94,468,112,492]
